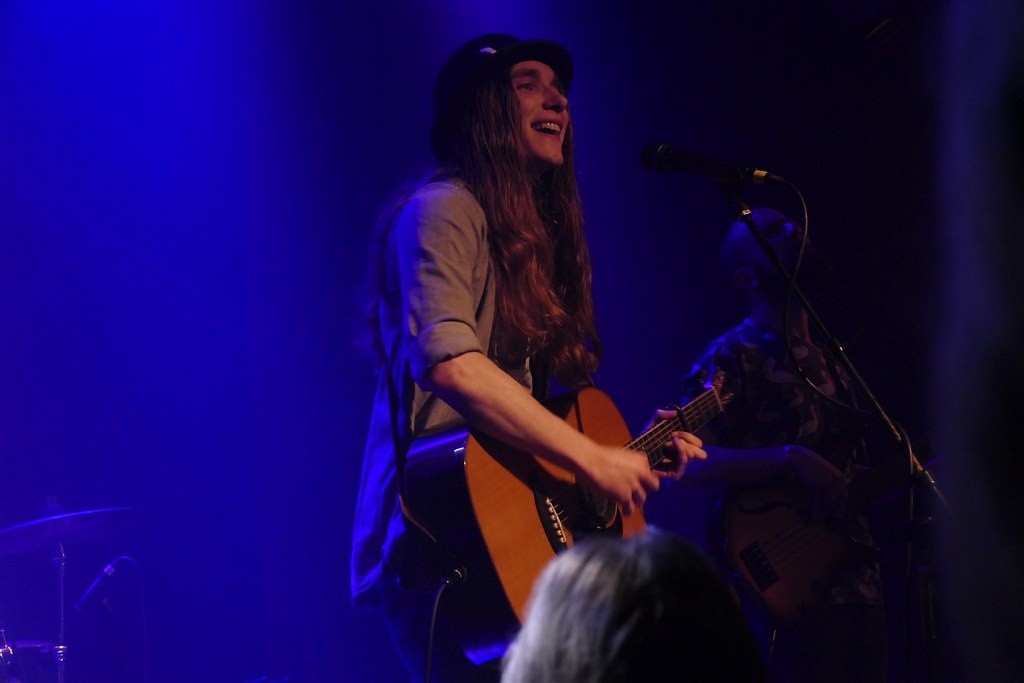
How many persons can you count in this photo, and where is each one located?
[349,31,709,682]
[649,206,946,680]
[500,531,777,681]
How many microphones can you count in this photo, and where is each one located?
[68,559,120,615]
[643,143,784,188]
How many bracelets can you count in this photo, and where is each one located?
[909,465,935,503]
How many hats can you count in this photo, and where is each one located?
[430,32,576,165]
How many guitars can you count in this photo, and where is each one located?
[401,371,739,667]
